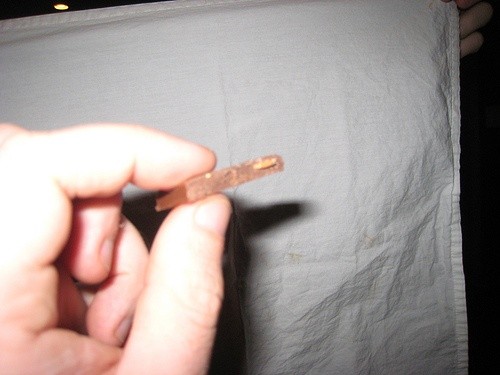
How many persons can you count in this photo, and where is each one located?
[1,121,230,375]
